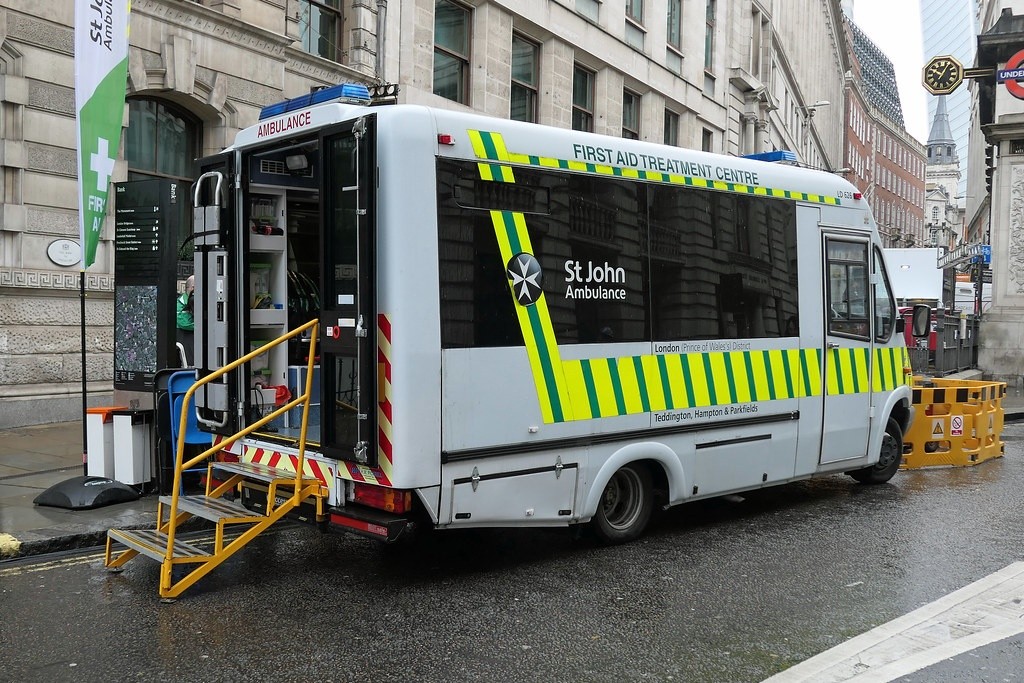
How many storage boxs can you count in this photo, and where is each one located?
[250,341,269,378]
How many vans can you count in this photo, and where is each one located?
[103,80,933,601]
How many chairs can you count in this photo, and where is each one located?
[168,370,221,496]
[152,368,195,495]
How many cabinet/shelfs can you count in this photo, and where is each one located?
[249,183,289,428]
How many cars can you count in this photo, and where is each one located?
[835,243,992,366]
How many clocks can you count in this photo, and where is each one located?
[922,55,963,95]
